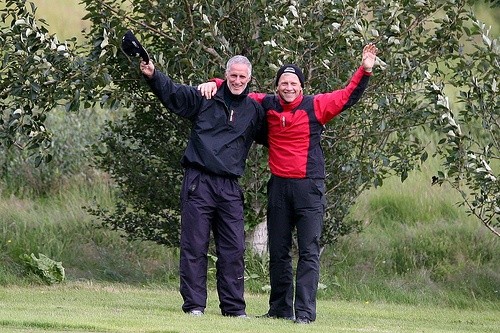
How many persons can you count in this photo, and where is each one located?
[139,54,268,319]
[197,42,379,325]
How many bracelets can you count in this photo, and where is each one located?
[363,66,372,72]
